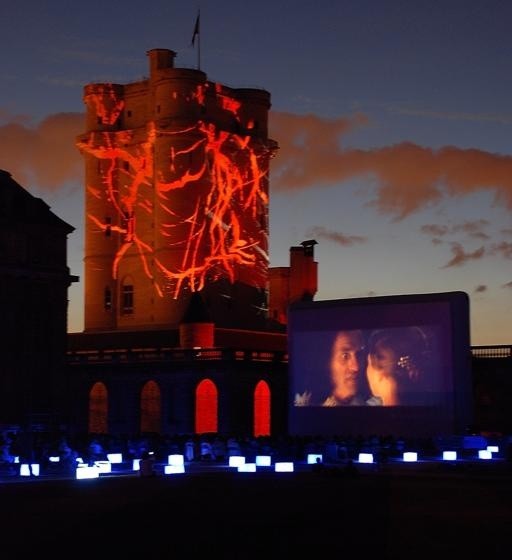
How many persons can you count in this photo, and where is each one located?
[292,328,368,408]
[365,328,426,407]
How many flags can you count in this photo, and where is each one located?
[191,15,199,47]
